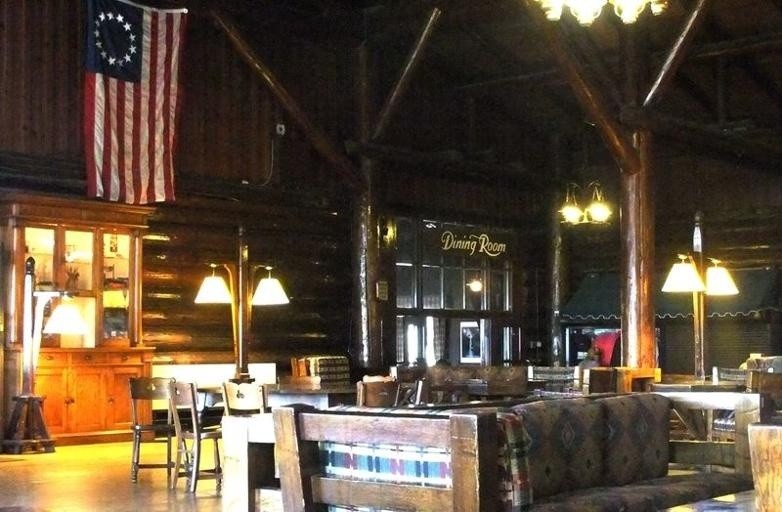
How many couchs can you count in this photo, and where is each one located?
[496,390,754,511]
[422,364,528,398]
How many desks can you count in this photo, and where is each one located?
[268,390,356,411]
[649,380,746,439]
[221,413,285,512]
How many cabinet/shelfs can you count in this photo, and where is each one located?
[0,187,157,448]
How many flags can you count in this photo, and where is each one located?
[83,0,190,205]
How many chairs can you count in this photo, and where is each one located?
[273,404,495,512]
[356,366,429,407]
[277,376,322,384]
[707,366,781,442]
[129,376,266,497]
